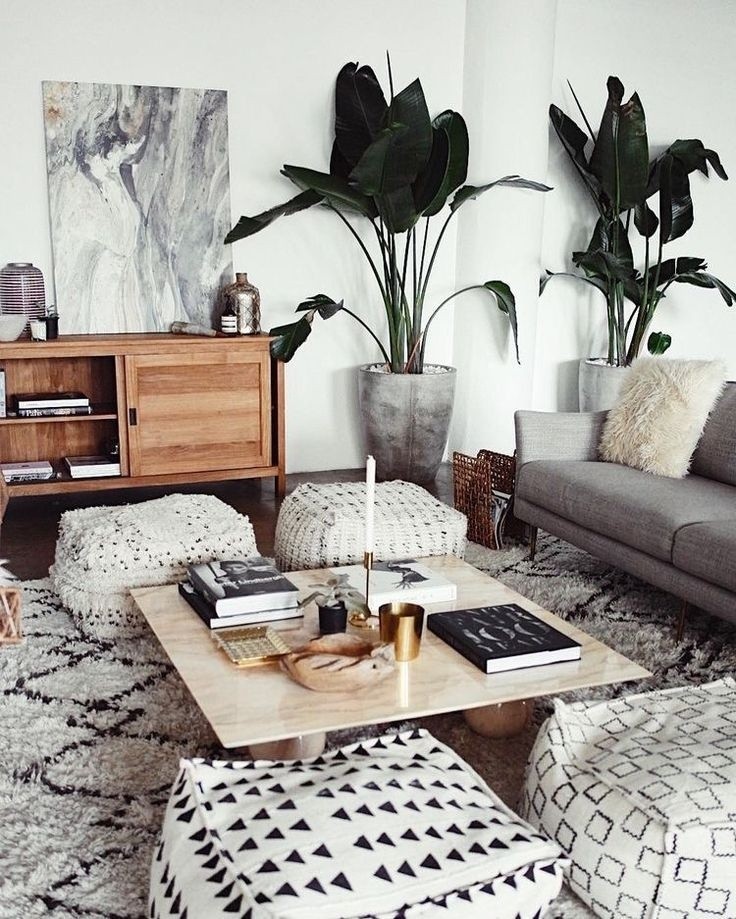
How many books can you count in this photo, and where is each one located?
[328,559,457,613]
[427,604,582,674]
[177,556,305,629]
[491,490,513,550]
[12,392,92,418]
[0,368,7,418]
[63,455,121,479]
[1,461,53,482]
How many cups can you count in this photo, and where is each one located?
[378,603,425,662]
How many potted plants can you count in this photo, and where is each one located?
[223,49,555,486]
[295,575,370,636]
[35,302,59,339]
[539,76,736,414]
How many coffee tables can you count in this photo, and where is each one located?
[129,552,653,763]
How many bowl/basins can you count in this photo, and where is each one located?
[0,314,29,341]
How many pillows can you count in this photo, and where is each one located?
[598,355,729,480]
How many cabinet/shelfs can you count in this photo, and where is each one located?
[0,330,286,498]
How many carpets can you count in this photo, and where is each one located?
[1,525,736,919]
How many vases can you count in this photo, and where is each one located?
[222,273,262,335]
[0,261,46,339]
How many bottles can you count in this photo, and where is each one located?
[222,272,261,335]
[1,264,46,338]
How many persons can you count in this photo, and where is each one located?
[216,560,299,596]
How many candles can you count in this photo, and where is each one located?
[365,455,376,552]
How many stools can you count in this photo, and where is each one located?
[517,676,736,919]
[147,729,572,919]
[275,480,469,573]
[48,494,261,640]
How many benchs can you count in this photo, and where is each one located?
[514,381,736,642]
